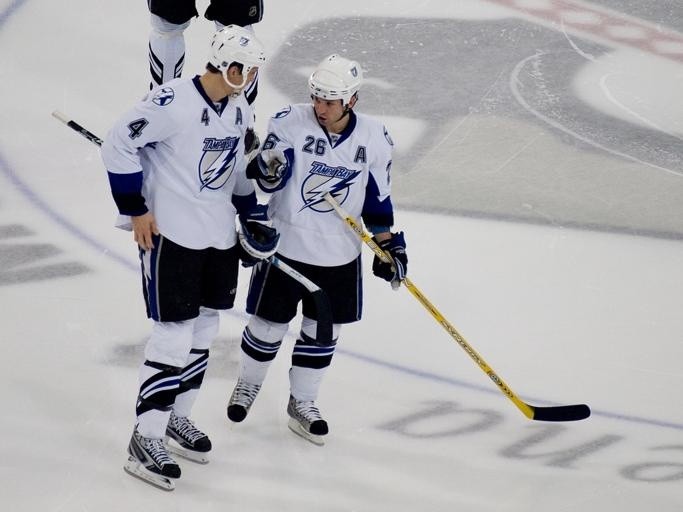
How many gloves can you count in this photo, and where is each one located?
[236,205,281,268]
[255,133,293,194]
[371,231,407,290]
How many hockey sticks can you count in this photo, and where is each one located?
[322,193,591,421]
[51,109,333,346]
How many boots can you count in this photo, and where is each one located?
[166,409,212,453]
[127,423,182,479]
[287,394,328,435]
[228,379,261,423]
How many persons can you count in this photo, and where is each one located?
[225,51,408,437]
[97,21,281,484]
[113,0,263,233]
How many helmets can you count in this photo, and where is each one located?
[208,25,267,90]
[308,54,363,106]
